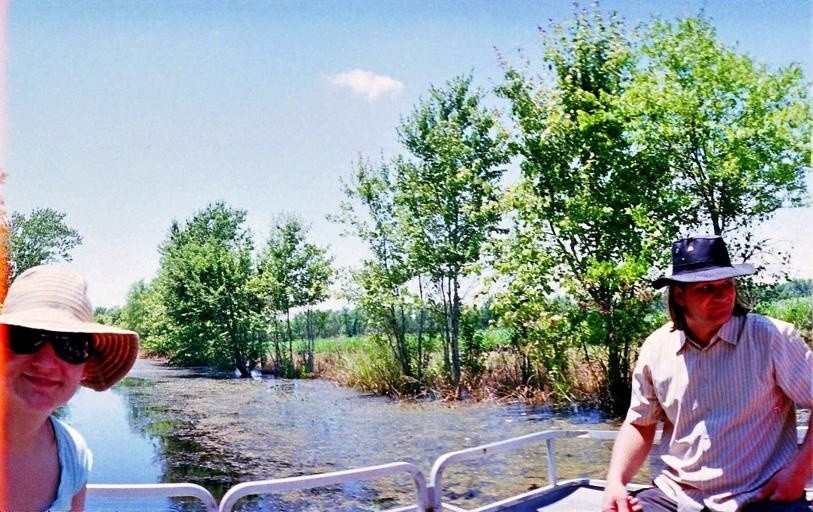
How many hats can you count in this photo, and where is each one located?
[0,263,140,391]
[652,237,757,291]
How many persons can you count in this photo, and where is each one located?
[0,261,139,512]
[599,235,812,511]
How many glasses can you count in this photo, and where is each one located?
[8,324,97,365]
